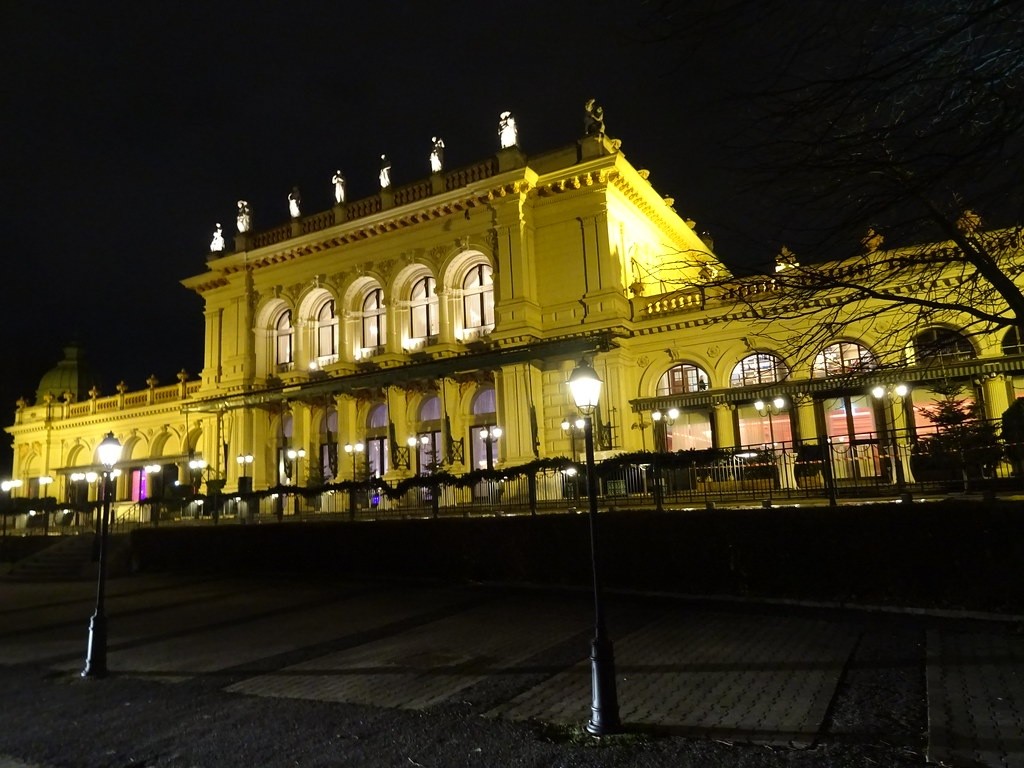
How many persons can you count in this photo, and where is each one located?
[498,111,519,148]
[237,200,252,233]
[332,170,346,204]
[430,136,445,172]
[210,223,225,251]
[288,187,301,219]
[378,154,391,189]
[584,98,605,135]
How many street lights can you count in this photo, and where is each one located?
[559,417,586,500]
[651,407,680,503]
[80,430,123,679]
[38,475,53,499]
[102,469,122,524]
[753,395,784,492]
[286,446,307,515]
[236,453,255,480]
[407,431,430,507]
[563,353,622,738]
[478,425,503,505]
[871,383,910,491]
[70,471,85,527]
[0,480,24,535]
[143,463,162,527]
[344,440,365,513]
[188,458,208,520]
